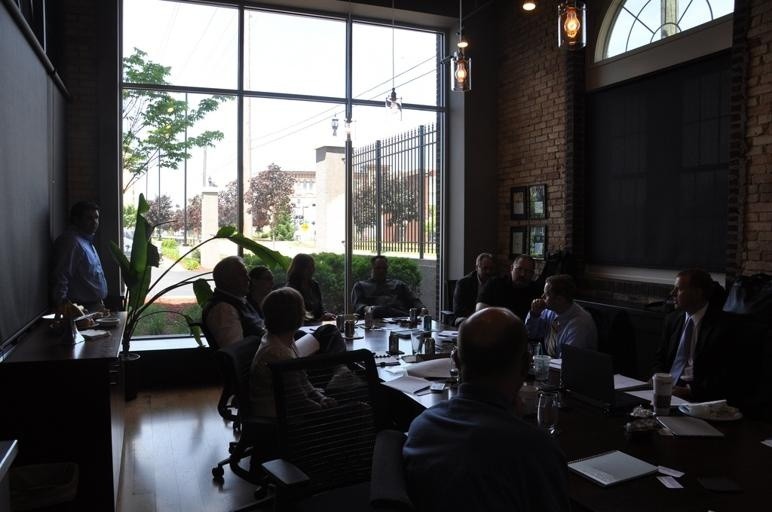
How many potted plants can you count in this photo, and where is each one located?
[107,193,291,401]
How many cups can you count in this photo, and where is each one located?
[537,392,560,429]
[411,330,423,355]
[336,313,358,337]
[650,372,674,415]
[532,355,552,380]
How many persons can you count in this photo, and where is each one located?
[49,200,109,310]
[398,307,573,511]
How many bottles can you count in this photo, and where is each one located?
[388,331,400,353]
[449,349,459,378]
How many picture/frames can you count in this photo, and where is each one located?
[510,186,527,220]
[527,224,547,262]
[509,225,526,259]
[527,184,547,220]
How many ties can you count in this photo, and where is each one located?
[667,315,701,389]
[547,315,561,357]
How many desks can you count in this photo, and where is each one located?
[0,310,129,512]
[297,316,771,511]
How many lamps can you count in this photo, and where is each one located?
[556,0,587,51]
[449,0,471,92]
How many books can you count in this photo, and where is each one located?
[567,449,658,488]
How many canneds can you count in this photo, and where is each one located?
[364,311,373,329]
[424,315,432,332]
[344,320,354,337]
[336,315,344,332]
[409,308,417,321]
[389,335,399,354]
[425,338,435,357]
[421,308,427,317]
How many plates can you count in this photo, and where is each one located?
[678,402,741,421]
[95,318,121,326]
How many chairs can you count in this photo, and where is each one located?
[210,335,328,499]
[265,349,402,511]
[189,317,241,421]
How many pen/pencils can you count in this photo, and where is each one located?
[452,333,457,335]
[310,329,317,332]
[414,385,430,394]
